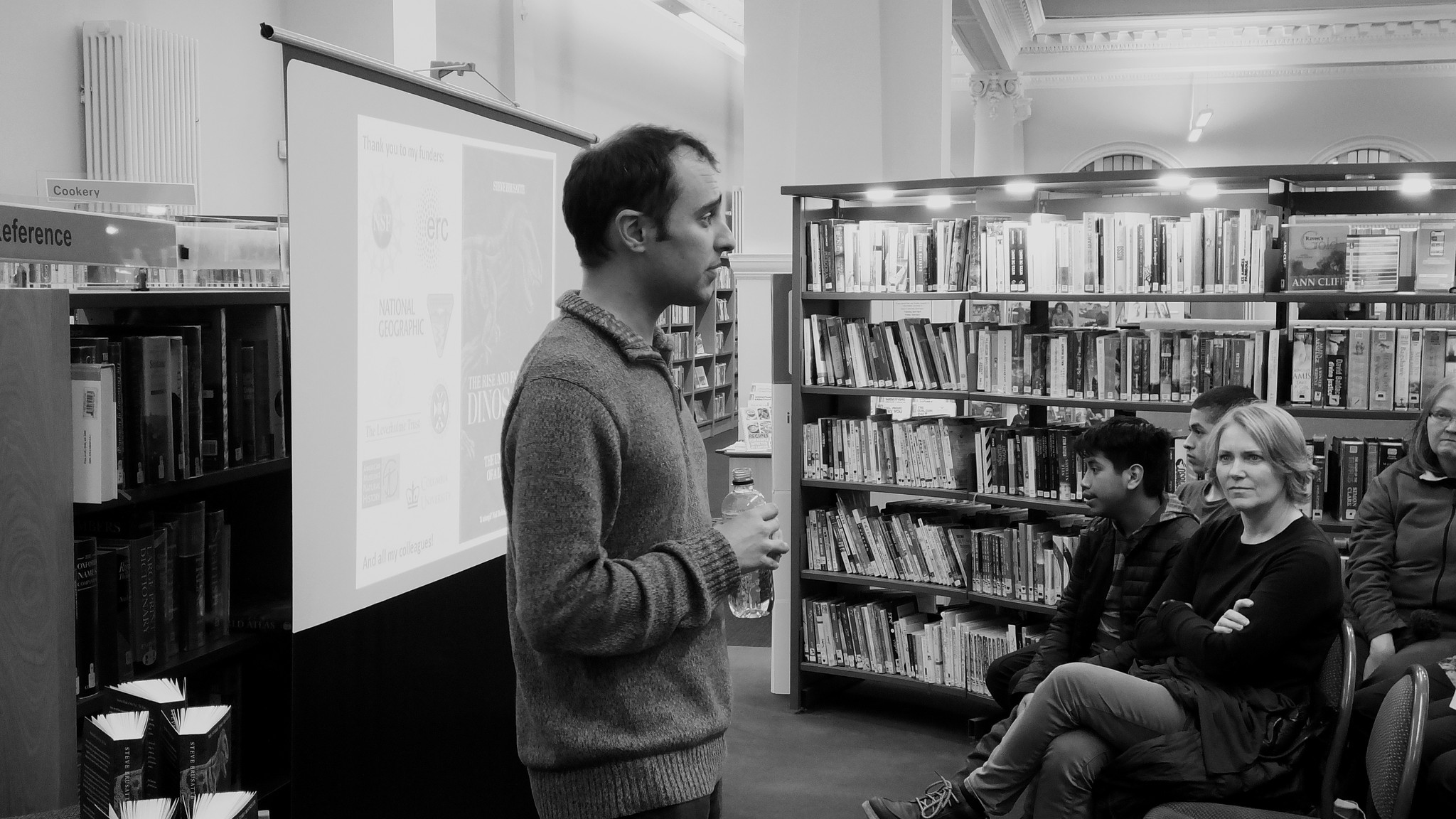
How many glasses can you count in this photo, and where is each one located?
[1429,409,1452,424]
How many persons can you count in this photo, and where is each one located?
[989,386,1259,708]
[861,404,1342,819]
[1345,373,1456,685]
[944,416,1202,781]
[498,126,790,819]
[1332,655,1456,819]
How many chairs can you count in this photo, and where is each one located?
[1144,663,1431,819]
[1319,619,1356,819]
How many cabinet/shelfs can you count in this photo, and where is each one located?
[0,287,289,819]
[660,256,739,440]
[780,161,1456,715]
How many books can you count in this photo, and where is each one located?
[804,209,1456,698]
[739,383,773,448]
[0,262,290,819]
[658,264,730,425]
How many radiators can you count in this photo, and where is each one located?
[82,20,202,215]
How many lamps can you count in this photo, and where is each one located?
[651,0,744,61]
[1187,82,1213,144]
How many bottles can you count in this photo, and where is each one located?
[721,468,774,619]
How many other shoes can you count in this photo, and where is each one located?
[862,769,990,819]
